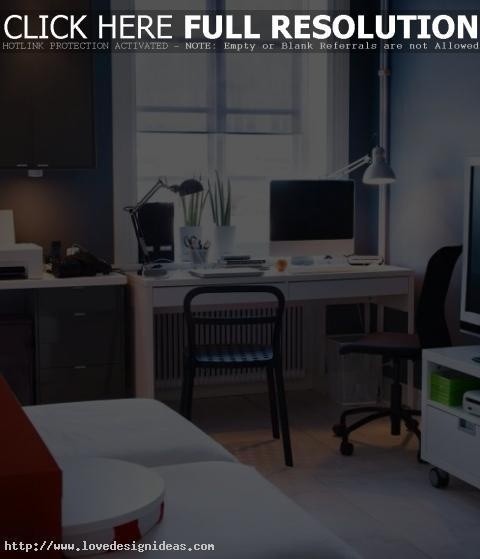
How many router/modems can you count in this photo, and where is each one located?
[349,254,384,265]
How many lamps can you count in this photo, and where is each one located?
[327,145,396,184]
[123,175,204,277]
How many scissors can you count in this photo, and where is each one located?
[184,235,202,250]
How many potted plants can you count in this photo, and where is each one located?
[181,171,210,262]
[206,167,236,257]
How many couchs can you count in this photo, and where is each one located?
[0,375,363,559]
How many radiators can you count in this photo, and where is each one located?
[152,298,312,402]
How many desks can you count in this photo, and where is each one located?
[114,257,415,407]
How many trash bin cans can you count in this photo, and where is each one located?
[327,334,384,406]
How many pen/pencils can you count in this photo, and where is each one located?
[198,240,211,250]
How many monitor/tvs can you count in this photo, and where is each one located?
[459,155,480,363]
[269,179,358,273]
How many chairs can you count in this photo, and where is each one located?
[182,283,295,467]
[333,245,463,457]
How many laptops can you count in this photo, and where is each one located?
[190,266,264,279]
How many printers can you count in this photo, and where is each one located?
[0,209,46,280]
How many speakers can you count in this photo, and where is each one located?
[137,202,175,264]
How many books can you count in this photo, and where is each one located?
[221,251,251,259]
[430,370,480,408]
[217,259,269,265]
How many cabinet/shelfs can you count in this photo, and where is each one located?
[419,344,480,492]
[1,316,34,405]
[35,286,127,405]
[0,0,99,175]
